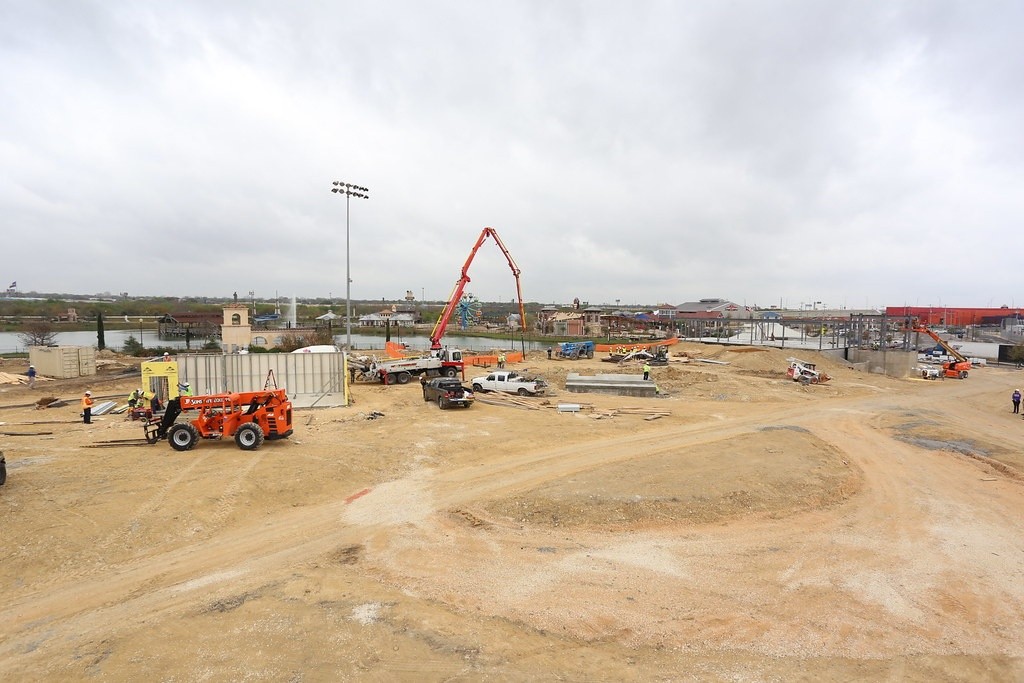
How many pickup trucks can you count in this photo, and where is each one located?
[472,371,545,397]
[423,377,475,410]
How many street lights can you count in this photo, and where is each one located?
[330,180,369,354]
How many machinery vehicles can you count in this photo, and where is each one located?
[898,326,972,380]
[355,226,528,385]
[616,344,669,368]
[78,388,294,451]
[555,341,595,360]
[291,344,370,373]
[787,358,831,384]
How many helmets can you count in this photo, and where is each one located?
[499,354,501,356]
[610,347,612,350]
[138,391,143,395]
[184,383,189,386]
[503,353,505,356]
[30,366,35,369]
[616,345,646,349]
[164,352,169,355]
[86,391,91,396]
[136,388,141,391]
[646,362,649,364]
[419,377,422,380]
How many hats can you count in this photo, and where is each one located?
[1015,389,1019,392]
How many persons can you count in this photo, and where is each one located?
[349,367,356,384]
[27,365,36,390]
[873,341,878,351]
[608,345,669,360]
[164,352,171,361]
[644,362,650,380]
[497,353,506,369]
[656,385,659,395]
[127,389,166,414]
[176,382,193,396]
[1011,388,1021,414]
[81,390,95,424]
[547,346,552,359]
